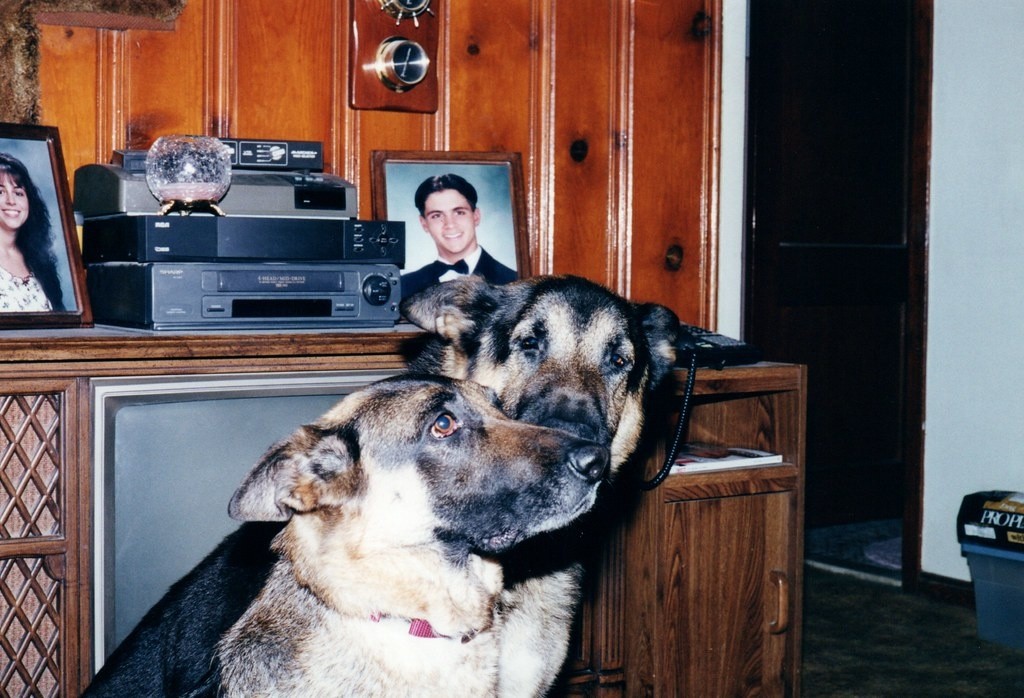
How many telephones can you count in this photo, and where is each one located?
[678,318,751,368]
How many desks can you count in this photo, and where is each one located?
[0,317,624,698]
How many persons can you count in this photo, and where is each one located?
[399,173,520,318]
[0,151,66,312]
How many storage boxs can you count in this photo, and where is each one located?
[961,543,1024,649]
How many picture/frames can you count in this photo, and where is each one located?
[368,148,532,321]
[0,123,97,330]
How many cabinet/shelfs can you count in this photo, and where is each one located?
[588,360,805,698]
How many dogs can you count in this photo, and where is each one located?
[399,274,684,698]
[74,371,612,698]
